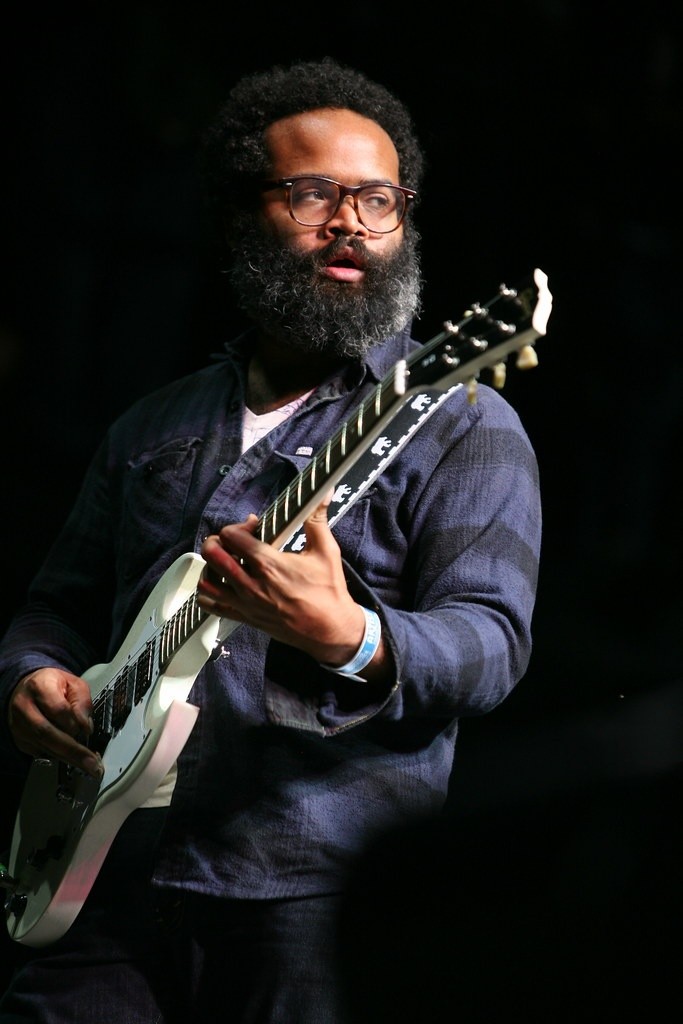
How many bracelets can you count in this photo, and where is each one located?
[311,604,383,679]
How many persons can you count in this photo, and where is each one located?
[2,61,543,1024]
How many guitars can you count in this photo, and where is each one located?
[3,264,555,946]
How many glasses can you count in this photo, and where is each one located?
[253,174,417,234]
